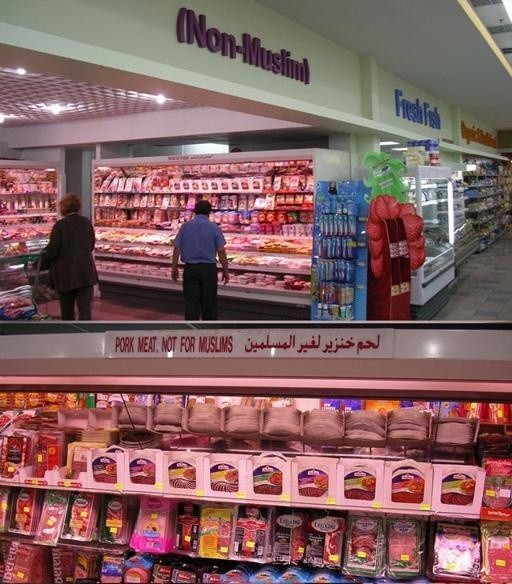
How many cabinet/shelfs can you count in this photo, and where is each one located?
[1,155,61,235]
[0,234,81,320]
[465,161,512,254]
[89,142,315,233]
[89,219,316,318]
[434,170,481,269]
[0,325,512,582]
[400,162,461,320]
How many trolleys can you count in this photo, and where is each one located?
[0,251,54,320]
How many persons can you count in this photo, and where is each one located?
[34,192,101,321]
[169,200,231,320]
